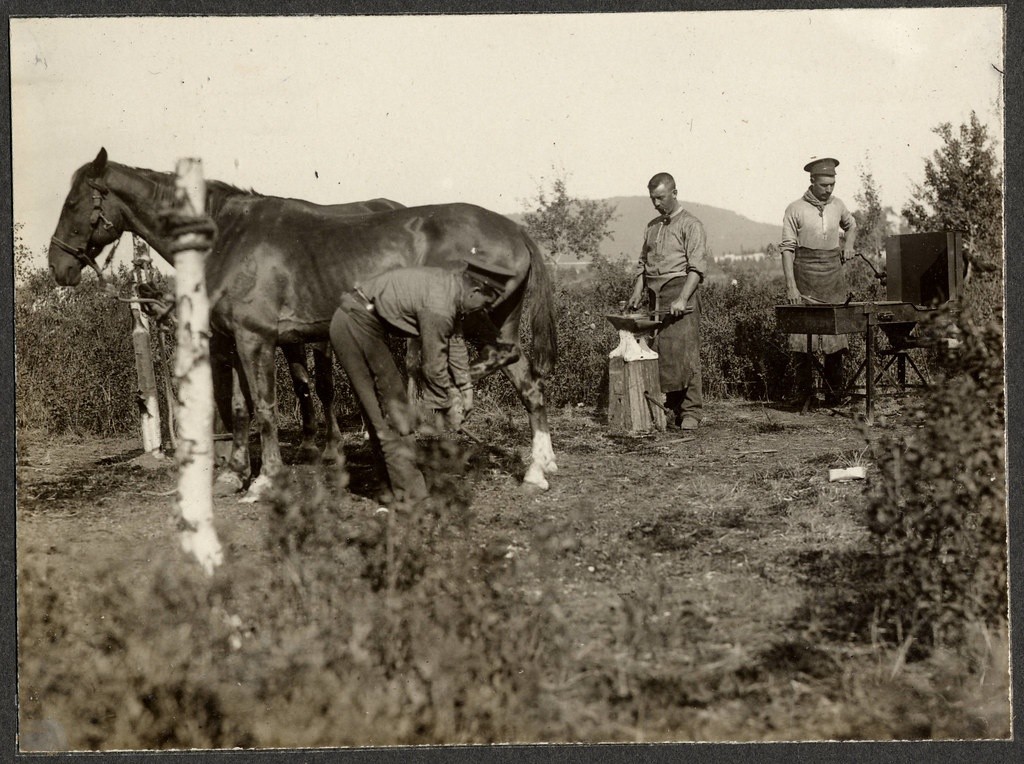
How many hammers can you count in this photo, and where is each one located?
[617,300,647,315]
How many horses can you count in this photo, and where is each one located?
[48,146,564,506]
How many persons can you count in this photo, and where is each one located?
[626,172,709,430]
[778,158,858,407]
[329,260,521,504]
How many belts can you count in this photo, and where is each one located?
[350,289,398,332]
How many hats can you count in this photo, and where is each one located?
[804,159,839,176]
[462,257,515,301]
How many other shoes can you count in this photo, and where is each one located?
[826,391,851,404]
[681,415,698,429]
[801,393,820,406]
